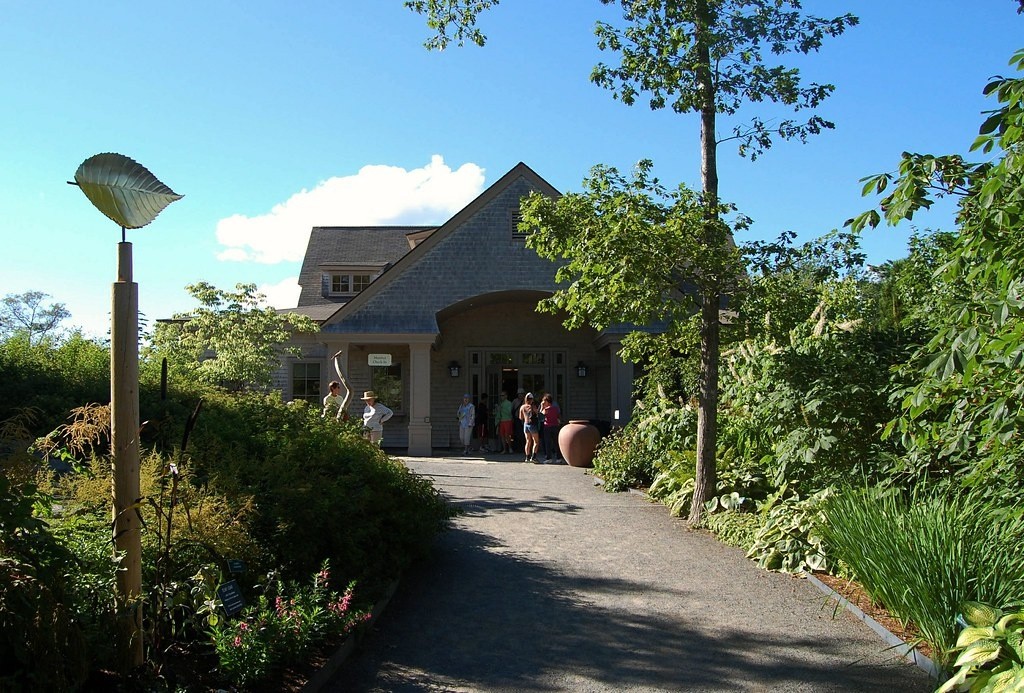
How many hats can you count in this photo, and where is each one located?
[526,392,534,398]
[360,391,378,400]
[516,388,526,394]
[463,393,471,399]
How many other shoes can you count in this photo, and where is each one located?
[463,448,473,457]
[479,445,513,454]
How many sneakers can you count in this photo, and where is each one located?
[524,455,562,465]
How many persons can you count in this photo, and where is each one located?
[495,391,515,455]
[457,394,476,456]
[322,380,349,422]
[511,388,527,453]
[360,391,394,451]
[536,393,563,464]
[518,392,540,464]
[476,393,492,452]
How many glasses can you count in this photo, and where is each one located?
[499,394,507,396]
